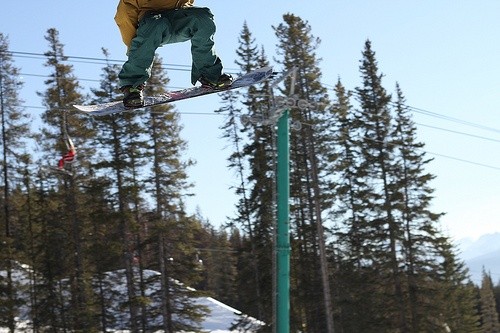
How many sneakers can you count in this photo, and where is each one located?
[198,75,233,91]
[120,83,145,107]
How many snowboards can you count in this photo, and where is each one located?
[72,66,274,117]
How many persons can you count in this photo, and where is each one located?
[113,1,231,108]
